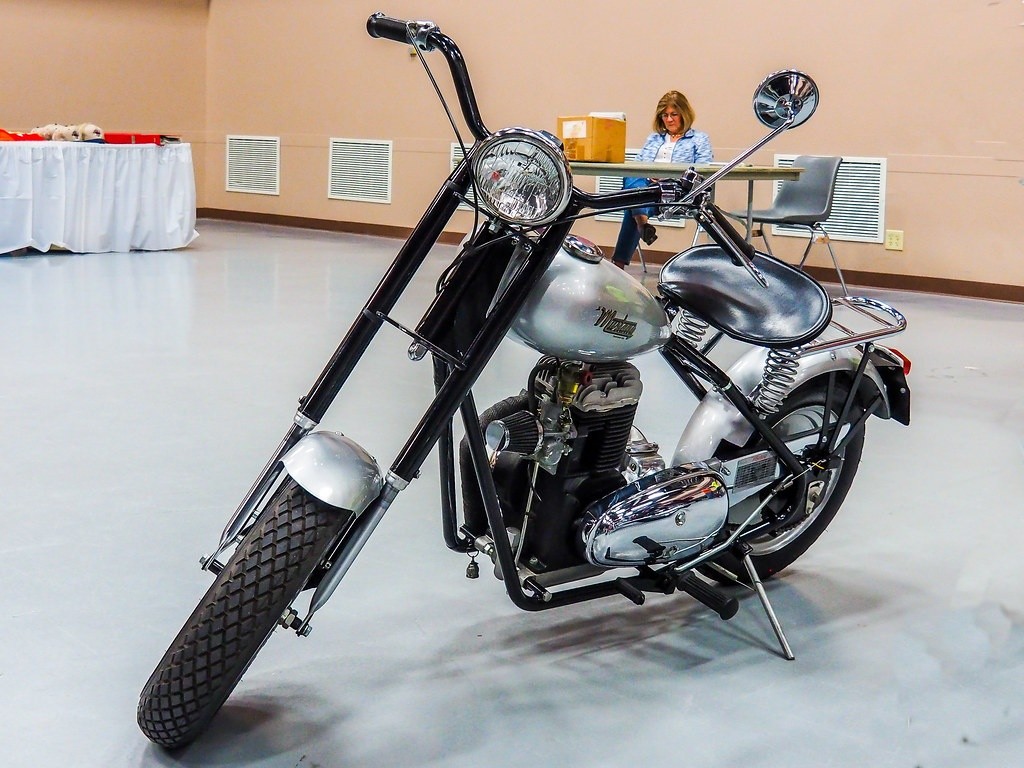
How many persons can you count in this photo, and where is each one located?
[608,89,714,270]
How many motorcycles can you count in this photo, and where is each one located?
[136,11,912,752]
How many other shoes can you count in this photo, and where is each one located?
[640,223,658,245]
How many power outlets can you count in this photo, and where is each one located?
[884,230,904,250]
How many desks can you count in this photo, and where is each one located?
[451,156,805,248]
[0,141,199,257]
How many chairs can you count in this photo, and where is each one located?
[732,156,847,297]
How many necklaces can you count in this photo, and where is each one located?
[667,129,681,139]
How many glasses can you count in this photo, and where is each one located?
[661,112,678,118]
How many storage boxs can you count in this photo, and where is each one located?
[558,116,627,163]
